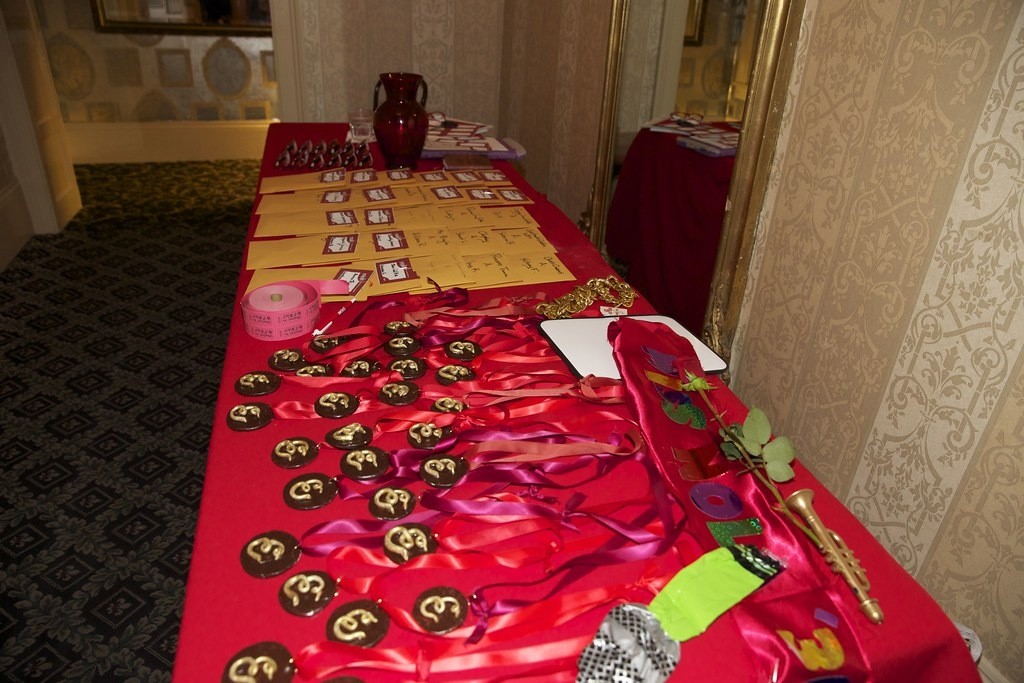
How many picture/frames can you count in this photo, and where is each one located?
[88,0,273,37]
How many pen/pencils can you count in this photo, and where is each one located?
[319,298,356,337]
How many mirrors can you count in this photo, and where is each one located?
[581,3,807,357]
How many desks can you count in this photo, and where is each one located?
[606,107,745,335]
[172,119,986,682]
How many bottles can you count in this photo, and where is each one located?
[372,72,430,170]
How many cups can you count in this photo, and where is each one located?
[346,103,377,141]
